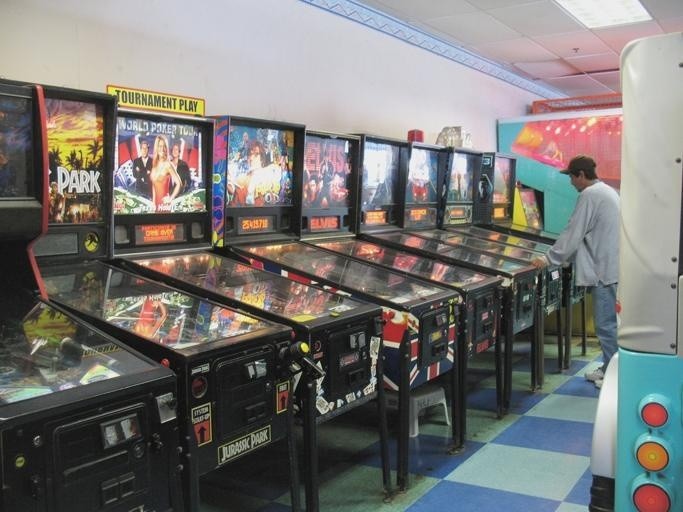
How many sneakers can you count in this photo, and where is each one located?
[585,367,604,382]
[593,378,603,388]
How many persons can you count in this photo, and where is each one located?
[531,154,618,387]
[132,136,153,198]
[211,283,273,335]
[303,151,352,207]
[118,295,187,347]
[149,135,183,213]
[227,132,291,208]
[170,142,192,196]
[49,182,64,223]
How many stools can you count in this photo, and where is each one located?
[382,385,452,439]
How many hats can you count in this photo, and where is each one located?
[557,153,596,175]
